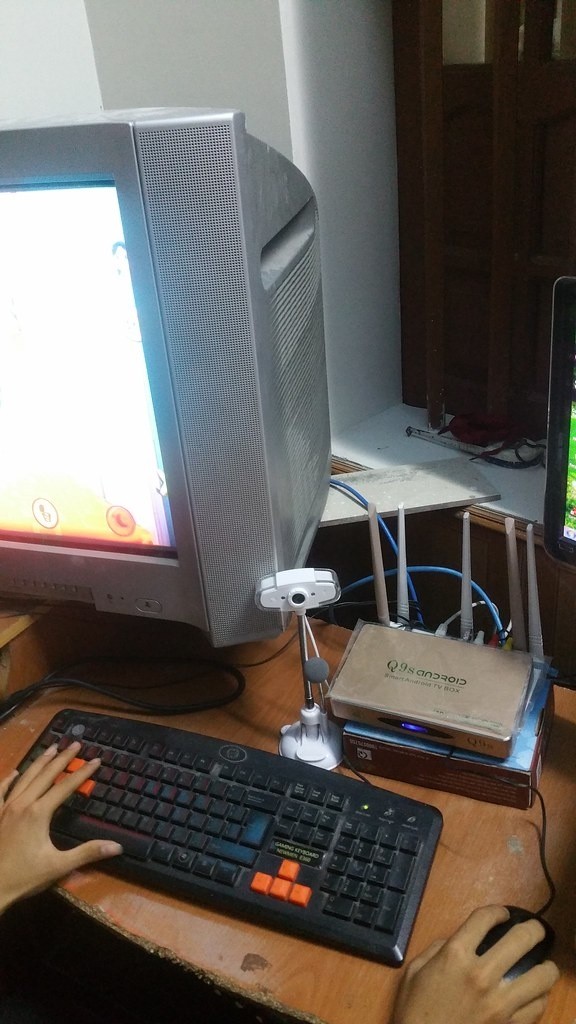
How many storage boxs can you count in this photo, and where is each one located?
[342,675,554,809]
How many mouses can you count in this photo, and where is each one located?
[474,905,555,988]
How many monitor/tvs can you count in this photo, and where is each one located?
[0,105,333,647]
[542,274,576,572]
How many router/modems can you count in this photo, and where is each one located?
[324,500,546,762]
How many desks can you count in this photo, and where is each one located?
[0,594,576,1024]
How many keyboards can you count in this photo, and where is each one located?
[5,709,445,967]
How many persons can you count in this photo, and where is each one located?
[0,741,560,1024]
